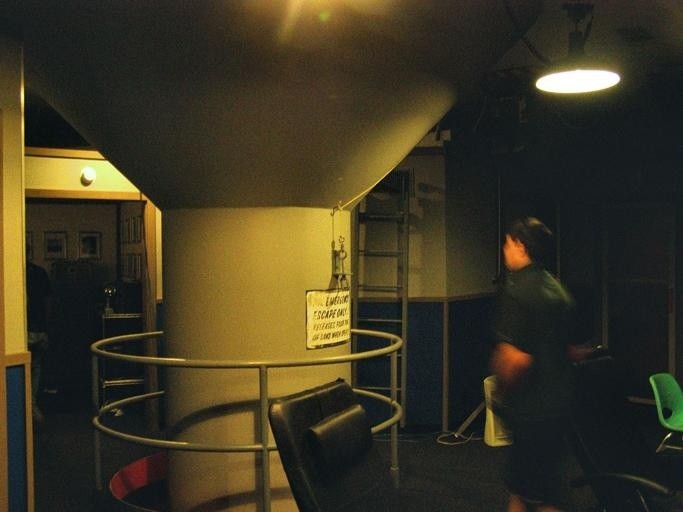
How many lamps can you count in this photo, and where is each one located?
[78,166,97,186]
[529,0,621,98]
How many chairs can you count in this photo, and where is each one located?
[554,360,683,509]
[644,366,683,455]
[266,372,479,512]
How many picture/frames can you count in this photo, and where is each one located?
[26,215,142,282]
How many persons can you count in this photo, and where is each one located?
[480,218,588,510]
[25,245,56,426]
